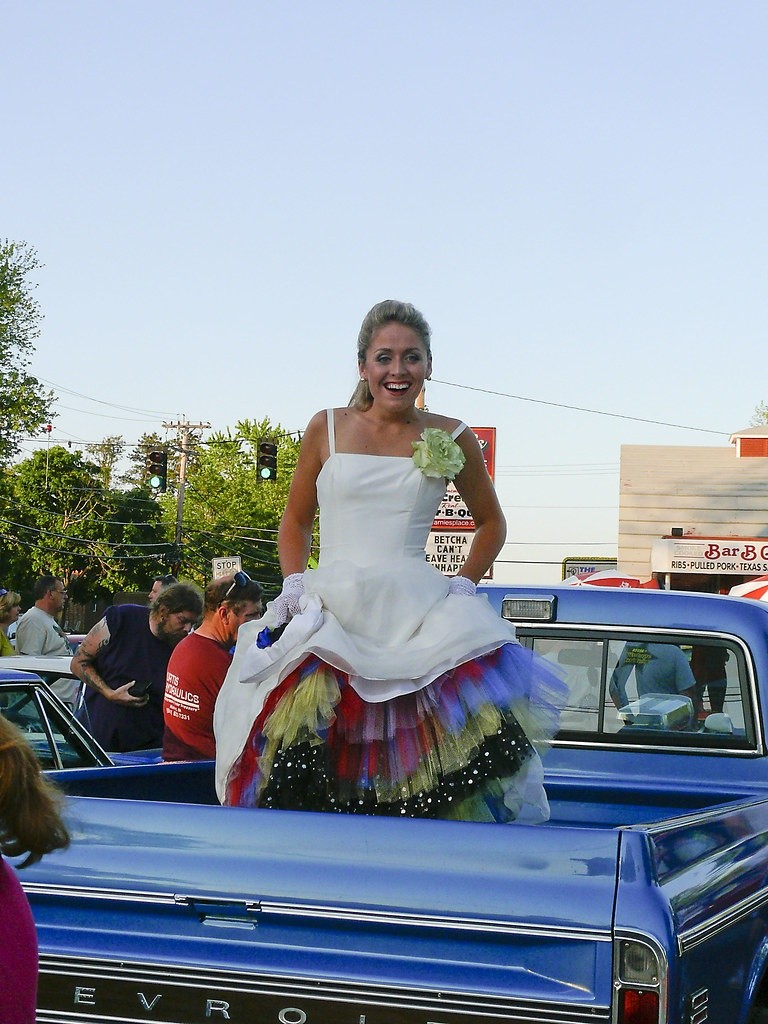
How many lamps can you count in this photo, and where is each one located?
[672,527,684,537]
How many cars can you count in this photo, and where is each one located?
[0,655,87,741]
[0,668,163,771]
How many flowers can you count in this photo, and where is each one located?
[411,428,468,482]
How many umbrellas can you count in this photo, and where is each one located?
[560,569,640,589]
[728,575,768,603]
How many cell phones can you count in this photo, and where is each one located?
[138,682,153,697]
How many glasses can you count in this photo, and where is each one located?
[54,589,68,595]
[165,574,171,583]
[217,570,252,608]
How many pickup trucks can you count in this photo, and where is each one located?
[1,585,768,1024]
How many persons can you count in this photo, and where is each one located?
[211,297,571,828]
[608,641,731,731]
[0,711,72,1024]
[70,569,264,763]
[0,574,74,658]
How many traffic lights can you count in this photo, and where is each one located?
[256,438,277,482]
[149,451,167,493]
